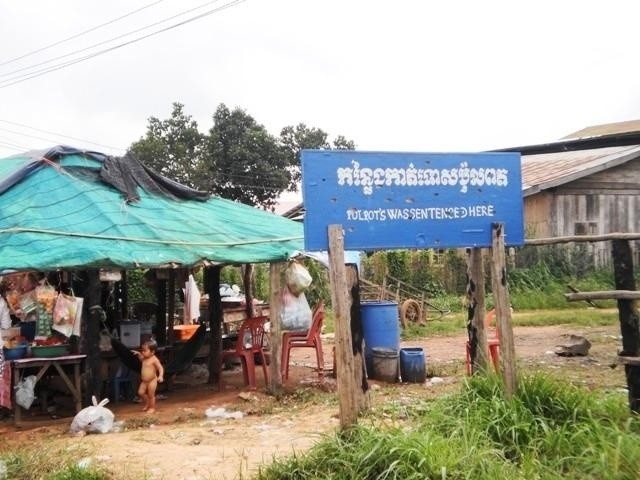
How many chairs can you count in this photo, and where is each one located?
[465,307,500,377]
[217,298,327,387]
[164,322,206,392]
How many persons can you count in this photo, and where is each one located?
[0,281,18,420]
[130,340,165,415]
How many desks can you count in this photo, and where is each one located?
[10,347,168,431]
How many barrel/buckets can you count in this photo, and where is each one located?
[371,347,399,382]
[400,348,425,382]
[361,300,401,374]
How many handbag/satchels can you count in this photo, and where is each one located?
[51,288,84,338]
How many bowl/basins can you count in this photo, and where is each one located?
[30,344,66,357]
[4,344,29,360]
